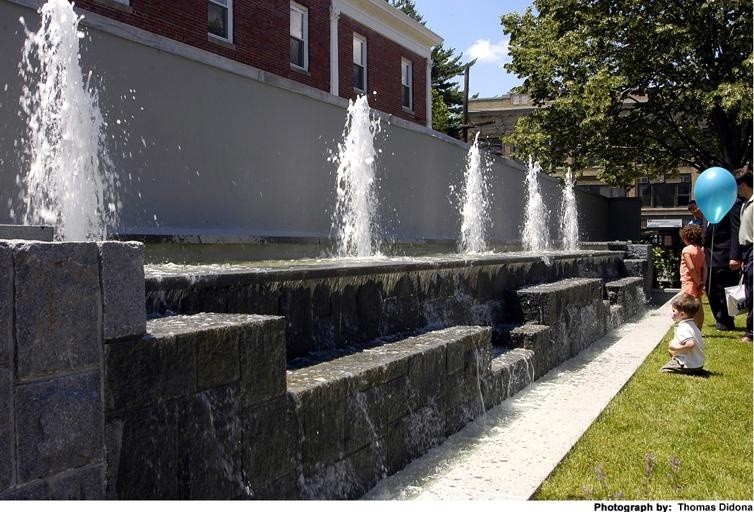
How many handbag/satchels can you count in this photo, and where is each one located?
[723,284,750,317]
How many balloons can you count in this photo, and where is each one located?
[694,167,739,225]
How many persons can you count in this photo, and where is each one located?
[688,200,704,227]
[679,224,709,330]
[705,198,741,332]
[660,292,710,377]
[735,170,753,342]
